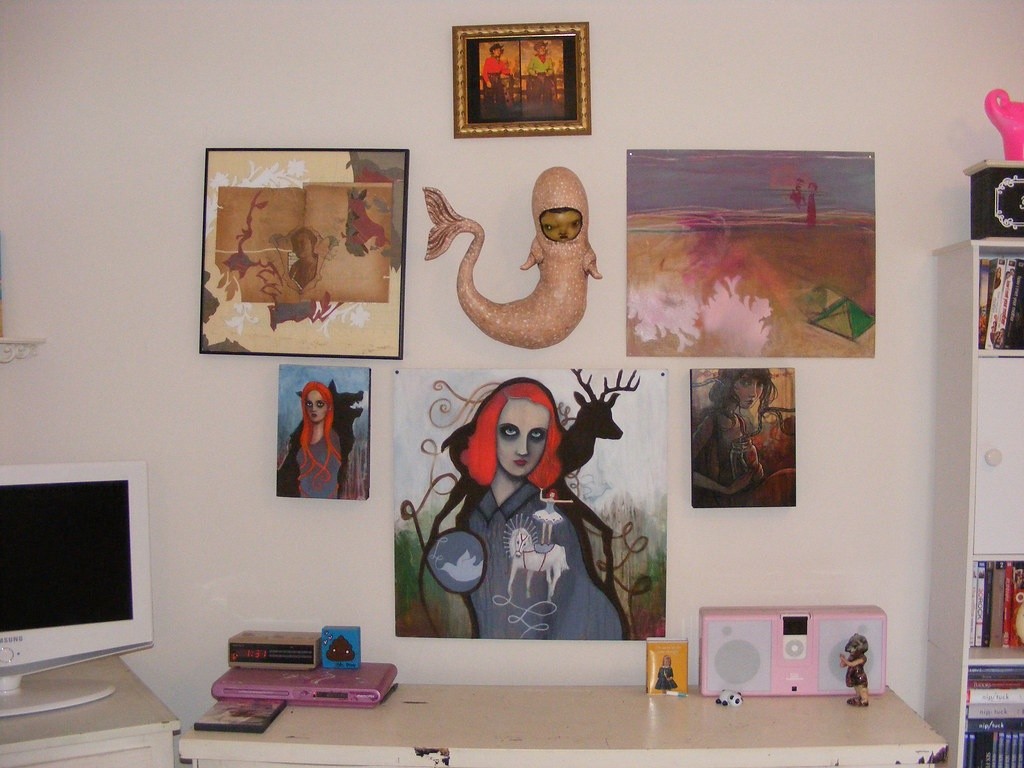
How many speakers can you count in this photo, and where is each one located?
[697,603,887,696]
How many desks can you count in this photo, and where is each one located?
[0,656,180,768]
[178,680,949,768]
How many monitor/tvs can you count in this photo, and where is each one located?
[0,462,155,716]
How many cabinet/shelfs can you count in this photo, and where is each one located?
[929,238,1024,768]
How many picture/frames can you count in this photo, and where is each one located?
[450,22,591,139]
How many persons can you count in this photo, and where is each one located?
[655,656,678,694]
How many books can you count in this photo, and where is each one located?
[962,665,1024,768]
[646,637,688,695]
[970,560,1024,649]
[978,257,1024,349]
